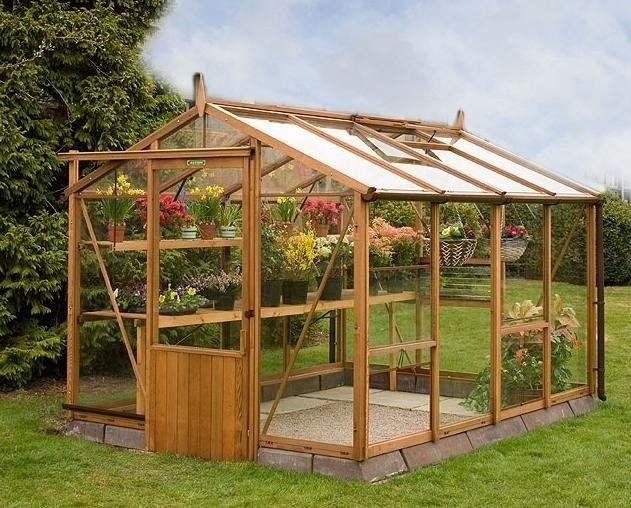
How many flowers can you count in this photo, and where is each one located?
[92,174,419,313]
[459,293,579,414]
[422,200,478,272]
[480,202,530,263]
